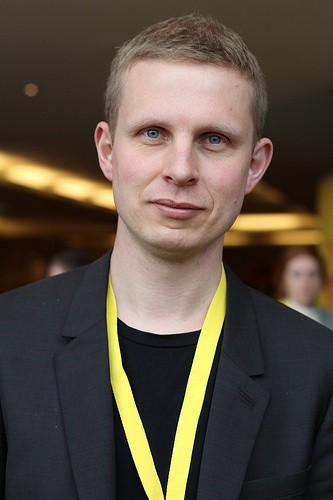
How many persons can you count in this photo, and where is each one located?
[0,15,332,499]
[274,247,333,328]
